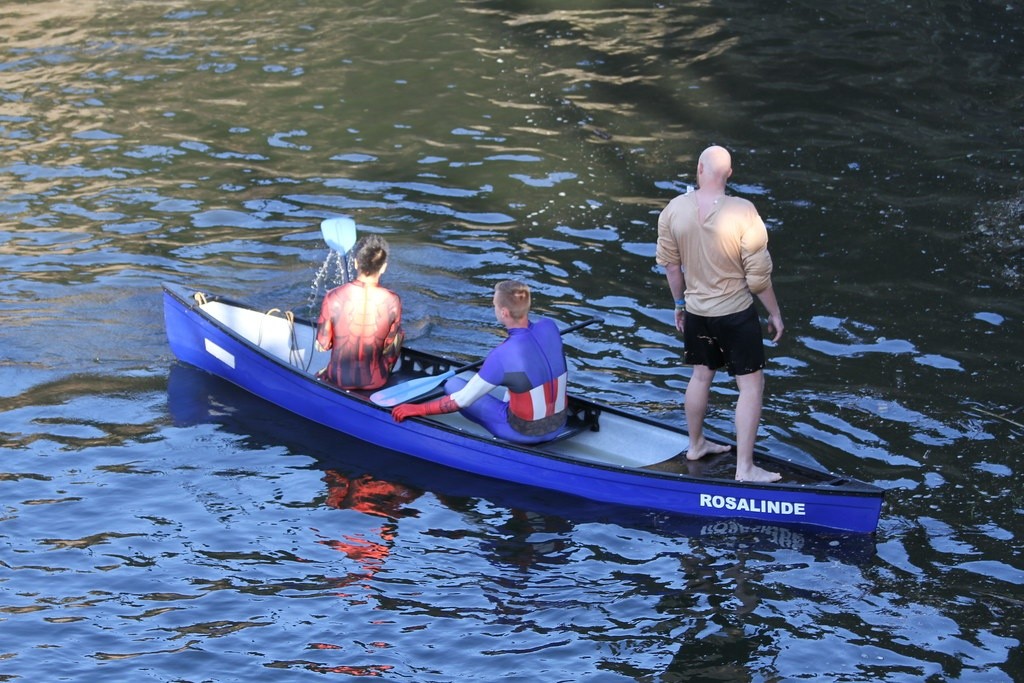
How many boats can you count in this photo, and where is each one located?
[162,281,885,539]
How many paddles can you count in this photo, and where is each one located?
[367,315,607,409]
[320,214,359,285]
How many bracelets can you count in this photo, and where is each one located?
[675,299,686,304]
[675,305,685,311]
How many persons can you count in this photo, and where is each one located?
[392,281,569,443]
[655,146,784,481]
[312,234,404,391]
